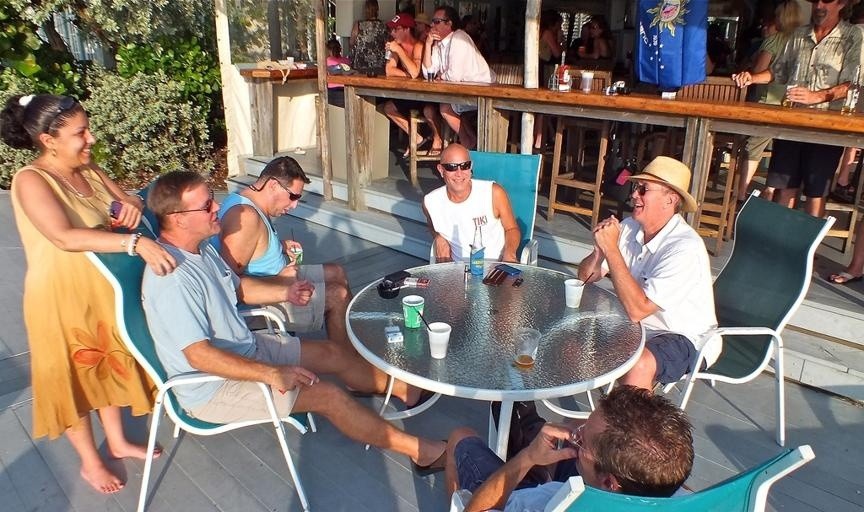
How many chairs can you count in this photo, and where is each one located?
[76,205,323,512]
[592,187,847,451]
[539,432,822,511]
[408,61,864,260]
[134,183,321,433]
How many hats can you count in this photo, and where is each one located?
[624,156,698,213]
[414,13,431,26]
[385,13,417,28]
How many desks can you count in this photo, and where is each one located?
[340,259,647,469]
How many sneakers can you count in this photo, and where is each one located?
[409,439,449,478]
[406,389,434,409]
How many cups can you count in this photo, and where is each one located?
[564,278,586,309]
[426,321,452,360]
[581,72,595,94]
[286,57,294,67]
[289,248,303,265]
[617,87,631,96]
[426,66,435,80]
[384,42,391,60]
[401,294,425,330]
[578,47,586,55]
[366,71,377,78]
[561,306,580,334]
[511,327,542,370]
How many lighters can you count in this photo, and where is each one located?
[278,389,287,394]
[512,278,524,288]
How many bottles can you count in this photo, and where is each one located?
[781,61,801,109]
[470,223,485,276]
[559,64,569,90]
[550,74,558,91]
[839,65,861,115]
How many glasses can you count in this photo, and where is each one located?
[166,189,214,216]
[431,18,448,24]
[391,27,400,33]
[806,0,838,3]
[568,424,588,458]
[441,161,472,172]
[47,95,79,128]
[274,177,302,201]
[632,183,647,196]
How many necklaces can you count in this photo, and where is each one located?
[43,158,105,217]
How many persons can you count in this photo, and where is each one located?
[444,385,696,512]
[140,172,449,477]
[540,11,618,70]
[421,143,524,391]
[326,0,501,160]
[209,156,395,398]
[0,93,176,495]
[706,0,864,284]
[564,155,722,397]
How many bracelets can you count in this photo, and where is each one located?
[128,233,142,257]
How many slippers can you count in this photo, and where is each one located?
[426,143,443,157]
[401,138,430,162]
[349,390,395,399]
[827,271,864,284]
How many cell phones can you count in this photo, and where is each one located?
[383,270,411,287]
[495,263,521,276]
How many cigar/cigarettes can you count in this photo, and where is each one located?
[310,380,314,386]
[392,284,409,290]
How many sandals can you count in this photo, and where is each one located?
[834,183,864,203]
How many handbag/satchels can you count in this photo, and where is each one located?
[491,400,546,457]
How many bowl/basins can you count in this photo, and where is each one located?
[377,281,400,298]
